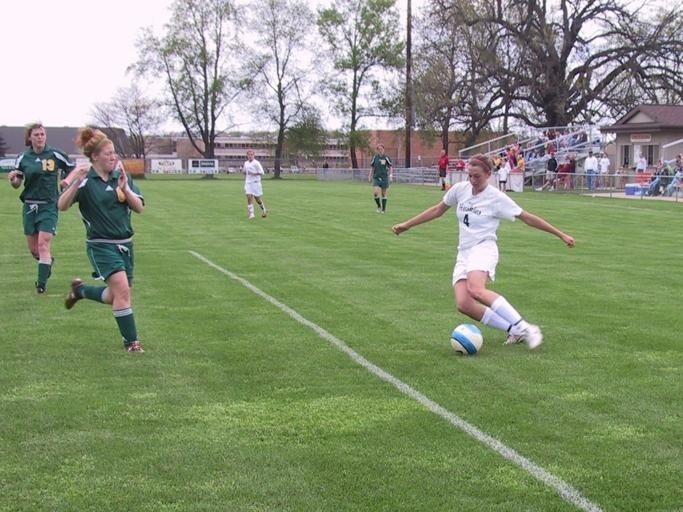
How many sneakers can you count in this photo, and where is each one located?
[124,340,146,354]
[48,257,54,278]
[520,325,543,350]
[263,211,267,217]
[502,326,524,346]
[66,278,84,309]
[35,282,47,294]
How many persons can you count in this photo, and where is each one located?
[241,150,268,222]
[57,128,144,354]
[8,123,77,293]
[438,129,683,199]
[368,142,392,215]
[391,153,576,350]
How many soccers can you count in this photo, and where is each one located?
[451,324,483,356]
